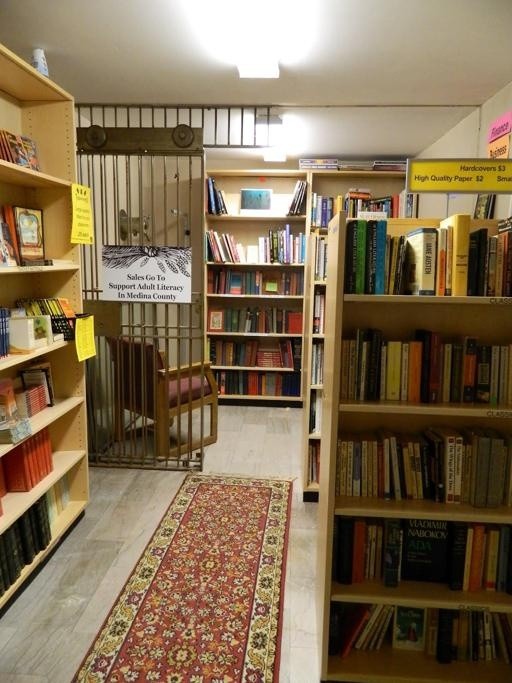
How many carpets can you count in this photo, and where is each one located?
[70,469,297,683]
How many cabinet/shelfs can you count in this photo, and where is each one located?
[0,42,90,619]
[204,170,512,682]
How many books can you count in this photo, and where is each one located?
[336,521,512,597]
[0,130,77,598]
[333,603,512,665]
[342,328,510,402]
[338,425,510,509]
[299,158,407,170]
[207,177,333,486]
[344,215,511,297]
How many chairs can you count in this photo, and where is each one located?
[106,336,218,462]
